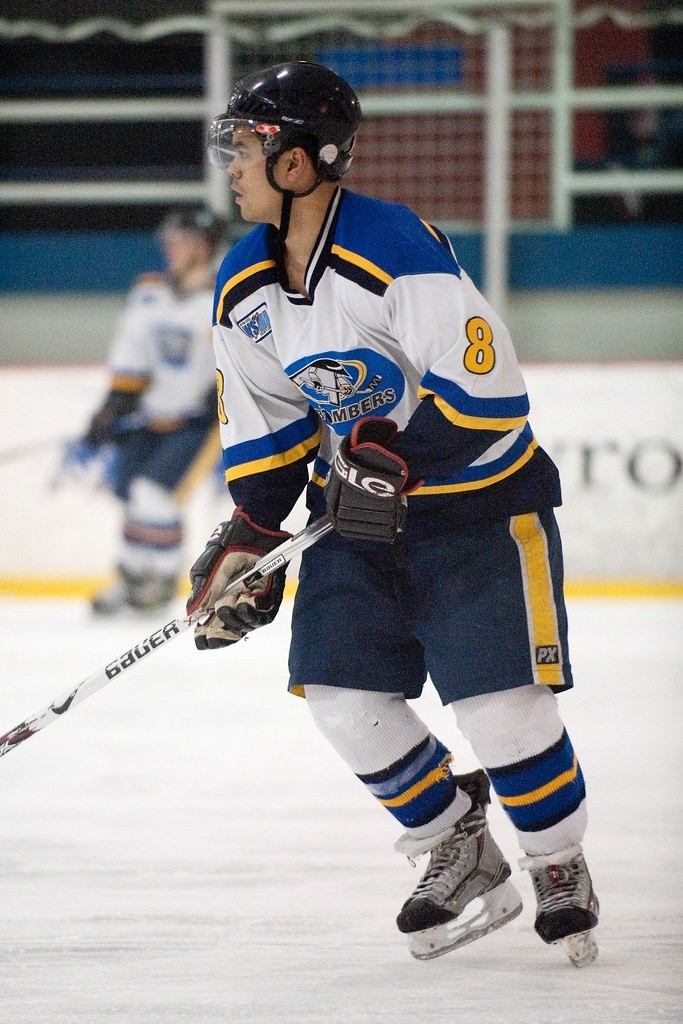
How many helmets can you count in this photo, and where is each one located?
[216,61,363,181]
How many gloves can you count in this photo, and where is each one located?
[324,418,422,546]
[188,511,295,650]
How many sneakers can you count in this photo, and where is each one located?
[392,770,521,961]
[92,570,177,616]
[521,846,600,969]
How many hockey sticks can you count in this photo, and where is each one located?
[0,506,332,777]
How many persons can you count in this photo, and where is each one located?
[184,60,613,942]
[82,204,239,617]
